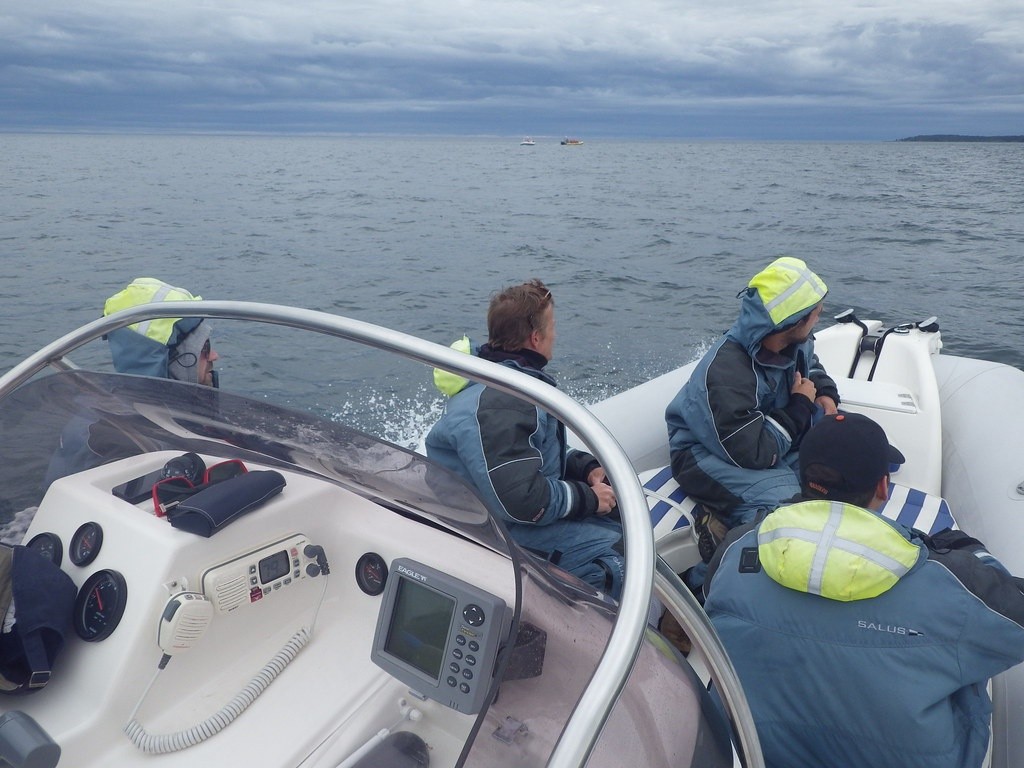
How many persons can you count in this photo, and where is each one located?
[665,257,842,595]
[38,278,219,511]
[699,413,1024,768]
[425,278,667,628]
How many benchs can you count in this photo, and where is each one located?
[635,464,703,572]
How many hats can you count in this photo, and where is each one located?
[0,541,78,694]
[798,413,905,495]
[169,323,211,392]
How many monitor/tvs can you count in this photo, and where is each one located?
[386,577,456,682]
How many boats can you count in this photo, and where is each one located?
[520,136,536,146]
[560,135,584,145]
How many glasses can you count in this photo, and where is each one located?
[536,286,552,311]
[153,458,248,517]
[202,341,211,360]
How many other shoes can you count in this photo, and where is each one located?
[658,607,691,657]
